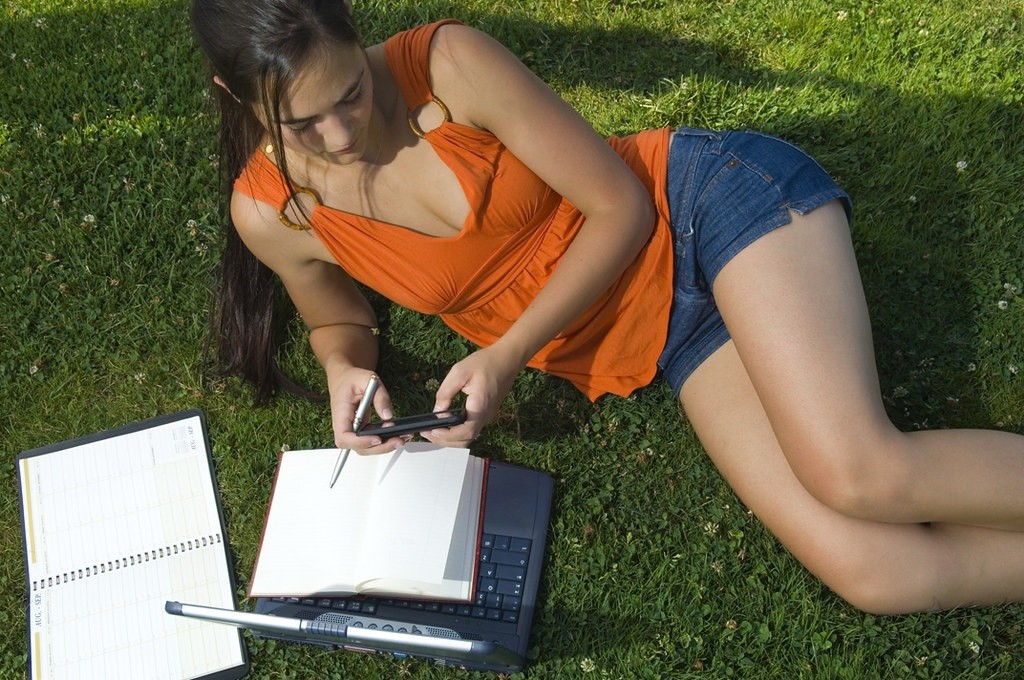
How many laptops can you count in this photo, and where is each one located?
[166,459,555,671]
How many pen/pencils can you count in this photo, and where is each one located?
[329,373,380,492]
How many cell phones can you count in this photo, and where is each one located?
[356,407,467,439]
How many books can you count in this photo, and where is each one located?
[246,441,491,606]
[16,406,249,680]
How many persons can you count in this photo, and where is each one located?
[191,0,1024,616]
